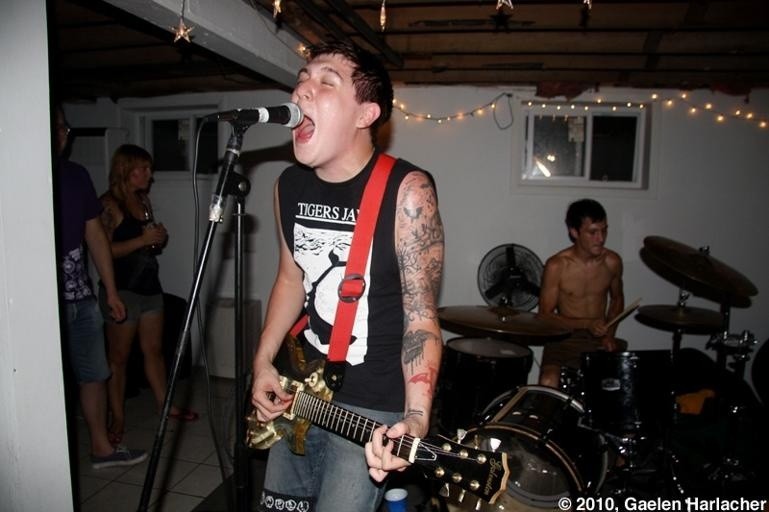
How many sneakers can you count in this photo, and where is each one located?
[88,446,149,470]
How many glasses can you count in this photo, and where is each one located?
[51,124,71,137]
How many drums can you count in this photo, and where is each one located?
[452,384,607,511]
[419,336,533,447]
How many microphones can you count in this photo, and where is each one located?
[209,101,304,129]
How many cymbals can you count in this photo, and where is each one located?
[436,305,576,343]
[634,304,727,335]
[640,235,759,309]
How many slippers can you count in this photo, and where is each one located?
[108,428,125,445]
[154,408,198,423]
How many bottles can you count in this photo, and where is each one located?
[142,209,162,257]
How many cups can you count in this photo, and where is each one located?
[383,487,409,512]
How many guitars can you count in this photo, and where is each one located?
[246,332,510,505]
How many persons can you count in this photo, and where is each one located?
[49,100,149,472]
[536,198,626,396]
[247,36,448,511]
[94,144,199,445]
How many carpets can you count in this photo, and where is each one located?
[188,450,267,512]
